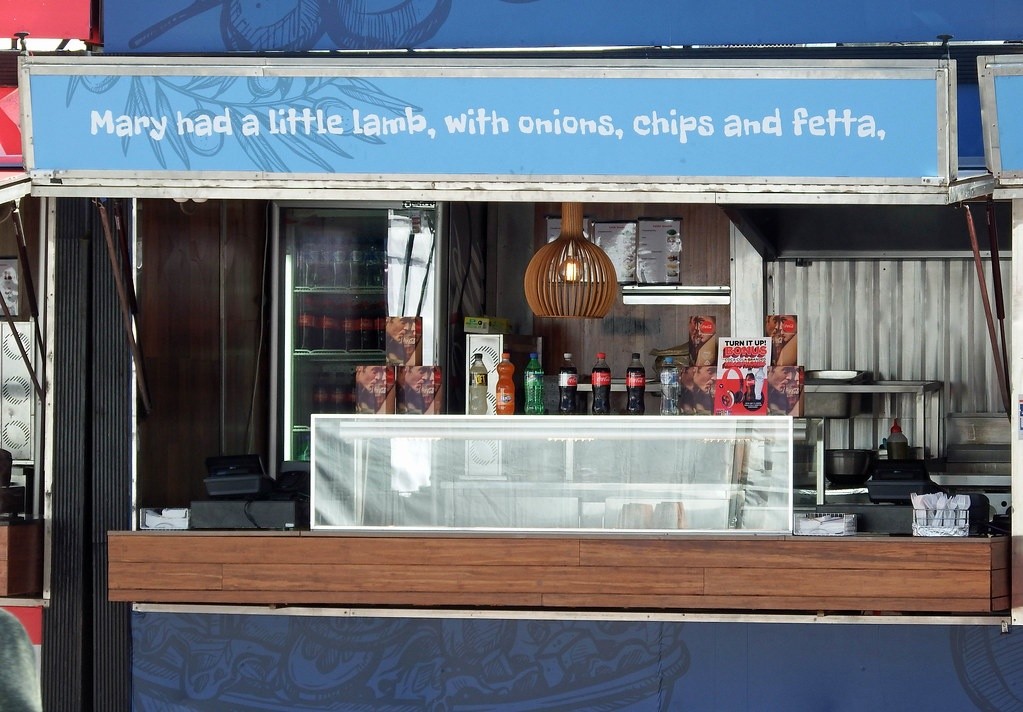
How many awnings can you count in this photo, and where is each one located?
[0,51,1023,202]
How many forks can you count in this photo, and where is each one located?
[911,492,971,527]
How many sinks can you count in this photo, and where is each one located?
[802,369,872,418]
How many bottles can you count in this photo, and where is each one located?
[626,353,645,415]
[523,352,545,415]
[297,242,384,287]
[591,353,611,415]
[298,296,386,349]
[469,354,488,415]
[886,419,909,460]
[496,352,516,415]
[557,352,579,415]
[659,356,680,415]
[313,372,357,410]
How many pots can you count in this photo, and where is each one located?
[824,449,876,484]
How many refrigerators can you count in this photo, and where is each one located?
[267,201,388,479]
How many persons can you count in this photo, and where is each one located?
[678,314,803,416]
[355,316,442,414]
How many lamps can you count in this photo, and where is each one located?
[523,202,617,319]
